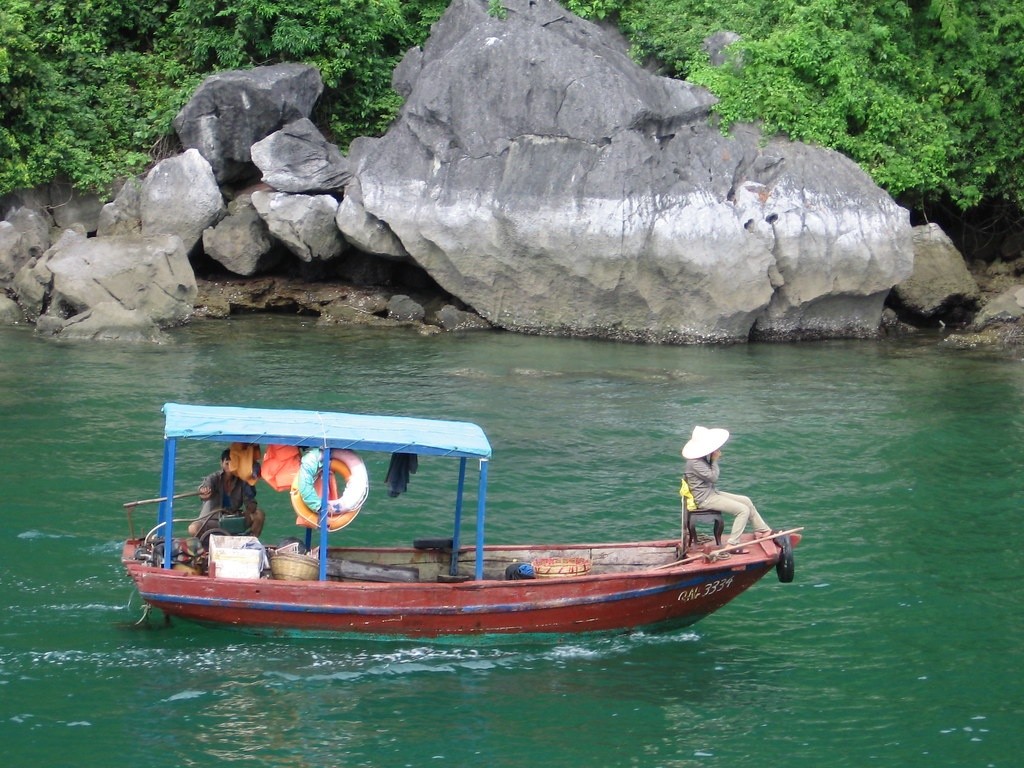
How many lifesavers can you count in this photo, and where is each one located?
[292,446,370,532]
[775,534,794,584]
[414,537,454,551]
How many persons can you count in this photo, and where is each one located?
[187,448,265,554]
[681,424,780,554]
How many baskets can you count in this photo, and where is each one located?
[269,551,320,581]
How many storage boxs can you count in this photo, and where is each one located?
[209,534,263,578]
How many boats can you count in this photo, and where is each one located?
[121,403,802,639]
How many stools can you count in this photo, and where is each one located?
[688,508,723,547]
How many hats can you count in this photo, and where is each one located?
[682,426,730,459]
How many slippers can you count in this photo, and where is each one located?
[769,528,784,536]
[730,548,750,554]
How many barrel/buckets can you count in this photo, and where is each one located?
[531,557,590,579]
